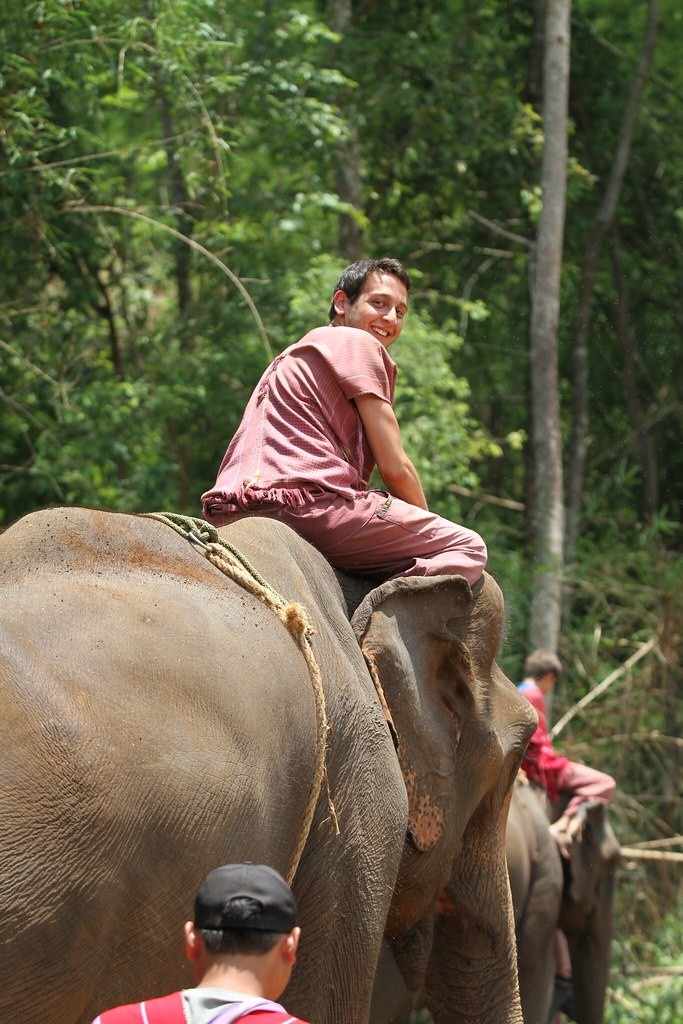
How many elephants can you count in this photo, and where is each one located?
[3,504,624,1022]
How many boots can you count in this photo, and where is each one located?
[548,814,582,858]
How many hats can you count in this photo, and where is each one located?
[195,860,297,932]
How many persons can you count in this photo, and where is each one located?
[515,647,616,859]
[89,860,310,1024]
[199,258,488,588]
